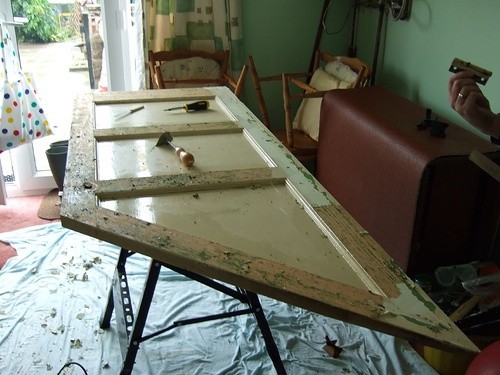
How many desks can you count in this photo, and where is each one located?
[54,82,484,375]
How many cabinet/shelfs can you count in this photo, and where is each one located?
[314,84,492,283]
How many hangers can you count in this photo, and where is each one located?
[0,19,25,29]
[13,15,29,24]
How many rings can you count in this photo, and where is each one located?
[458,93,465,101]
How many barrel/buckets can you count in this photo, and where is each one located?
[47,140,69,192]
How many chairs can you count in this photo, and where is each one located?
[146,47,229,90]
[283,49,372,175]
[146,48,251,101]
[238,45,369,172]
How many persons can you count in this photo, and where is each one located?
[449,69,500,151]
